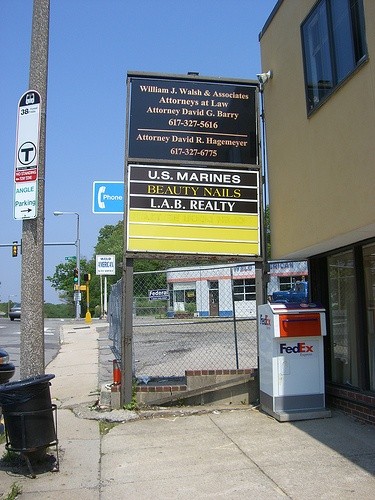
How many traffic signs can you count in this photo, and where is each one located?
[13,179,38,220]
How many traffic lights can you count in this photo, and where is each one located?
[84,273,91,281]
[73,269,78,282]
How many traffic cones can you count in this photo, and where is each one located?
[108,358,121,387]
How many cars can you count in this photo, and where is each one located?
[8,303,21,321]
[0,347,15,384]
[271,281,308,305]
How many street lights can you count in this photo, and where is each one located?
[7,295,17,319]
[53,211,81,319]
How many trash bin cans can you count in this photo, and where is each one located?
[0,374,55,448]
